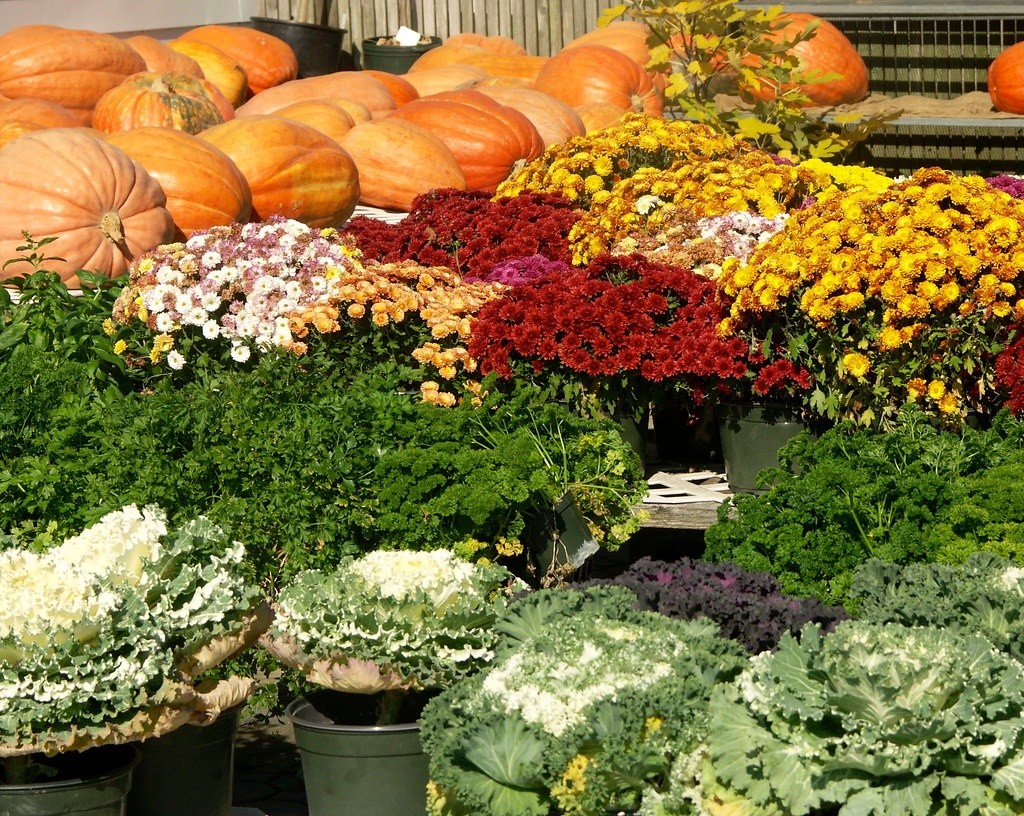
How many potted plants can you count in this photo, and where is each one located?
[124,515,276,815]
[259,546,530,816]
[1,501,228,815]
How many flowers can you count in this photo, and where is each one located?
[418,583,750,816]
[696,618,1024,816]
[0,111,1024,655]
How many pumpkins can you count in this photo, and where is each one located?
[0,13,1024,291]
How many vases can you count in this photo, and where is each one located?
[361,34,442,74]
[250,15,347,79]
